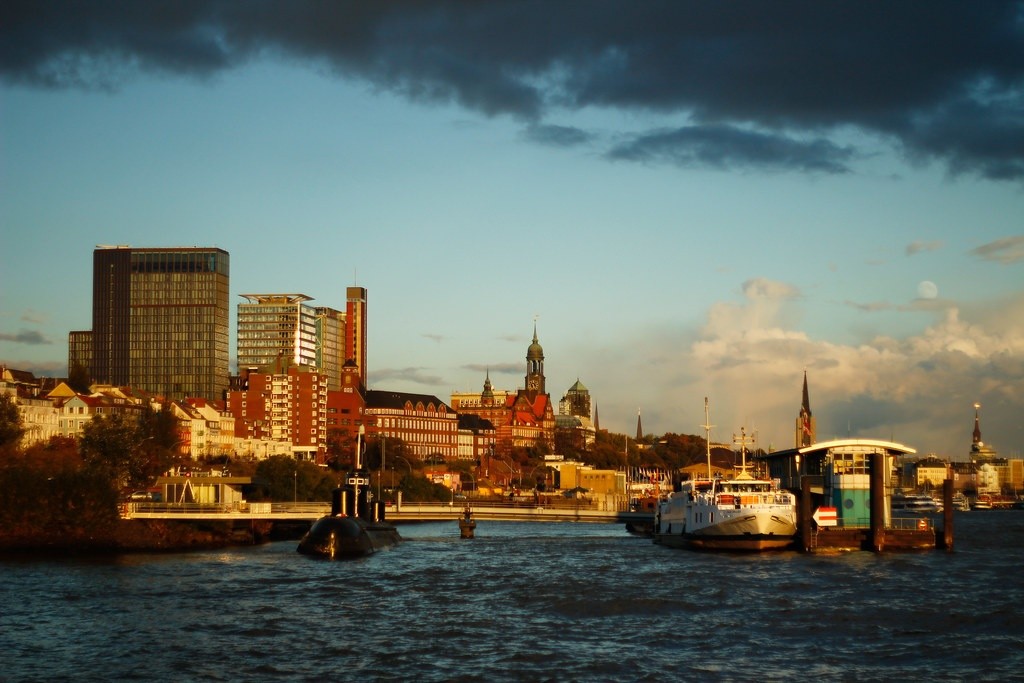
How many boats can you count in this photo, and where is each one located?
[652,479,802,555]
